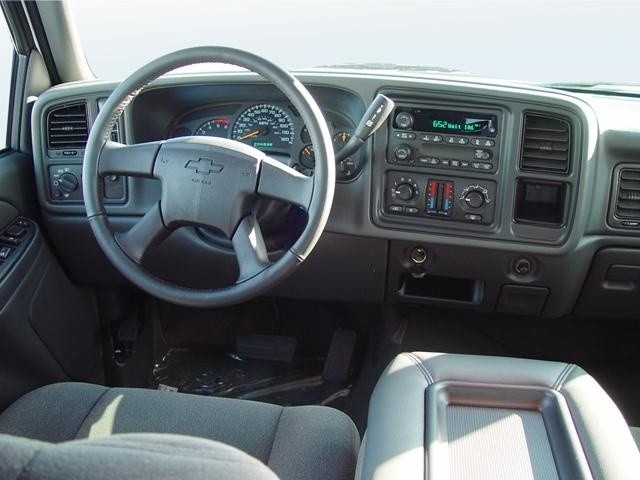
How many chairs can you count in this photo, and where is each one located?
[0,380,360,479]
